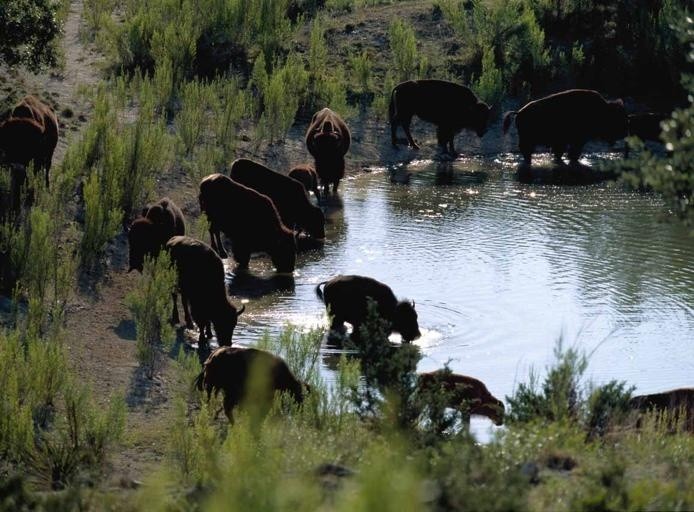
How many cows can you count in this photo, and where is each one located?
[388,79,494,158]
[165,236,245,348]
[315,274,419,350]
[413,372,505,436]
[191,344,311,425]
[501,88,679,164]
[125,194,185,265]
[305,107,351,193]
[610,388,694,435]
[196,156,327,273]
[0,95,59,195]
